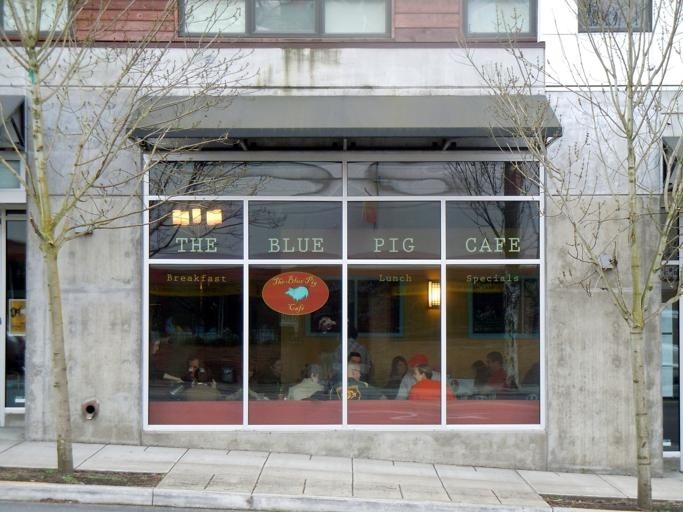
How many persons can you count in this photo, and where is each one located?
[327,326,389,401]
[225,356,325,402]
[149,332,212,385]
[467,351,519,401]
[383,353,457,401]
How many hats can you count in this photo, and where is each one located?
[408,355,426,367]
